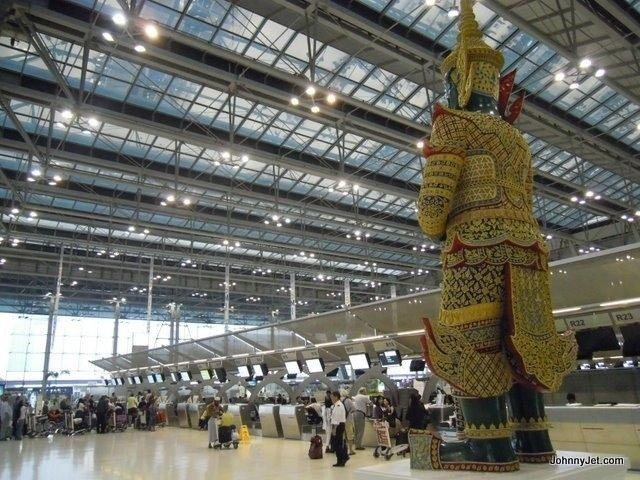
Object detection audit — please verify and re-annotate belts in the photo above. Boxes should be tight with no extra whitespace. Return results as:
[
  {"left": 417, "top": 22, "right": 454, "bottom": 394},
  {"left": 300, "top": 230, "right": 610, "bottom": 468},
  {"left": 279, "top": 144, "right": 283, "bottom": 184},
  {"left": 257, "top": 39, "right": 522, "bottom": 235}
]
[{"left": 351, "top": 410, "right": 366, "bottom": 416}]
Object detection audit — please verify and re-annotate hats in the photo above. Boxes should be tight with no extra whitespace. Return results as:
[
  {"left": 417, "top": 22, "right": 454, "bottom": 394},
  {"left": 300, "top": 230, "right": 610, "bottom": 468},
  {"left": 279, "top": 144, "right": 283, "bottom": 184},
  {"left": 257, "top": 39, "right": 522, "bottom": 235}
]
[{"left": 214, "top": 397, "right": 220, "bottom": 400}]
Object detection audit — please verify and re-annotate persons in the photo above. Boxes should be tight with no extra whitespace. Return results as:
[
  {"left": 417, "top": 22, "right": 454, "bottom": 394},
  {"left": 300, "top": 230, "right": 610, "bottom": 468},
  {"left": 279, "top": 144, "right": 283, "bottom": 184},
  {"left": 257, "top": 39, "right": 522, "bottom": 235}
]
[
  {"left": 275, "top": 386, "right": 456, "bottom": 467},
  {"left": 0, "top": 390, "right": 170, "bottom": 443},
  {"left": 407, "top": 0, "right": 579, "bottom": 473},
  {"left": 201, "top": 396, "right": 225, "bottom": 449},
  {"left": 225, "top": 397, "right": 243, "bottom": 448},
  {"left": 566, "top": 391, "right": 578, "bottom": 406}
]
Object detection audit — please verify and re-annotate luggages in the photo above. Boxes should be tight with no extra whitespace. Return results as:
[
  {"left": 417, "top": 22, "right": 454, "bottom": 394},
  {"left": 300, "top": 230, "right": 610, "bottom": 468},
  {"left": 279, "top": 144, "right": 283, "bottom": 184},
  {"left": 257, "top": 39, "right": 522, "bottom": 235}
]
[{"left": 218, "top": 425, "right": 238, "bottom": 442}]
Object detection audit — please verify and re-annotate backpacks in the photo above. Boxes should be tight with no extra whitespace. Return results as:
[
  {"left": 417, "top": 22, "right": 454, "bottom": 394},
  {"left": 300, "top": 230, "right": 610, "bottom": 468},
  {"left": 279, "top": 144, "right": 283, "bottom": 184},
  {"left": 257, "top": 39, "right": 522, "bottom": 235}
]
[
  {"left": 305, "top": 405, "right": 323, "bottom": 425},
  {"left": 308, "top": 435, "right": 323, "bottom": 459},
  {"left": 341, "top": 397, "right": 350, "bottom": 419}
]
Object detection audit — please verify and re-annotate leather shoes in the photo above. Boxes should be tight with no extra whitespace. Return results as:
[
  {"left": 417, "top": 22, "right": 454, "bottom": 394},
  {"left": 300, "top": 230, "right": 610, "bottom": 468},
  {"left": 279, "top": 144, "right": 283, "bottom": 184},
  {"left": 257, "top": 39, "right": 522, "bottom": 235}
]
[{"left": 332, "top": 456, "right": 351, "bottom": 467}]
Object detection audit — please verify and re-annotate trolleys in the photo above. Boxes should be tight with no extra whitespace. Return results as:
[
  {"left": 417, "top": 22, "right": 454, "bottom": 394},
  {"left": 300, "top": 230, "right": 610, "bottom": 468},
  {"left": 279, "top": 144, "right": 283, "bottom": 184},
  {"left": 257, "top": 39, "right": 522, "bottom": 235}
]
[
  {"left": 365, "top": 415, "right": 409, "bottom": 460},
  {"left": 213, "top": 416, "right": 239, "bottom": 450},
  {"left": 26, "top": 408, "right": 147, "bottom": 438}
]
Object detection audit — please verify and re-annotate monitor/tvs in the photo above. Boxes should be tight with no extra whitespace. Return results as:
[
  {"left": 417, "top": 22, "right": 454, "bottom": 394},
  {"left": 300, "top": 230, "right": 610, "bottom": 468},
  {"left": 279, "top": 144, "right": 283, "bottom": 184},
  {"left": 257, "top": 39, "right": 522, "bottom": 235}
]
[
  {"left": 377, "top": 350, "right": 402, "bottom": 365},
  {"left": 170, "top": 372, "right": 181, "bottom": 382},
  {"left": 348, "top": 353, "right": 371, "bottom": 369},
  {"left": 200, "top": 369, "right": 212, "bottom": 380},
  {"left": 103, "top": 373, "right": 165, "bottom": 386},
  {"left": 180, "top": 370, "right": 191, "bottom": 381},
  {"left": 574, "top": 323, "right": 640, "bottom": 352},
  {"left": 306, "top": 358, "right": 325, "bottom": 373},
  {"left": 285, "top": 360, "right": 303, "bottom": 374},
  {"left": 213, "top": 368, "right": 225, "bottom": 380},
  {"left": 252, "top": 363, "right": 268, "bottom": 376},
  {"left": 238, "top": 365, "right": 253, "bottom": 377}
]
